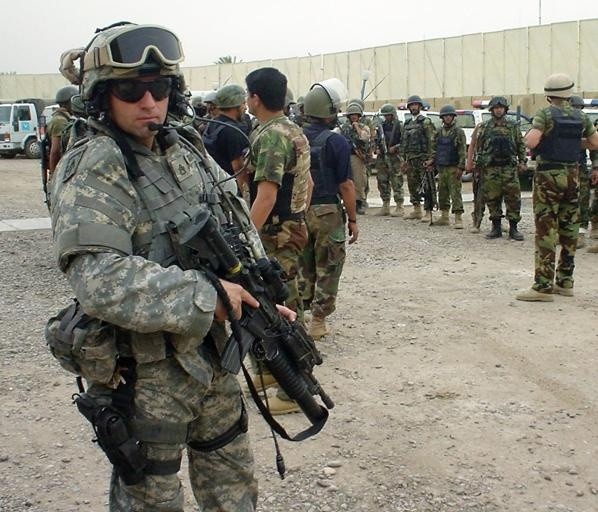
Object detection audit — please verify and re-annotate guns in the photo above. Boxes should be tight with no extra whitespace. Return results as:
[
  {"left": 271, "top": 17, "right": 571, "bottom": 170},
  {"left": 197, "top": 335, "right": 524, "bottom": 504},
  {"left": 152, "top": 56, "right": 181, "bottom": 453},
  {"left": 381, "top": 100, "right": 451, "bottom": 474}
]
[
  {"left": 336, "top": 120, "right": 371, "bottom": 158},
  {"left": 416, "top": 167, "right": 438, "bottom": 226},
  {"left": 178, "top": 210, "right": 335, "bottom": 441},
  {"left": 374, "top": 122, "right": 397, "bottom": 175},
  {"left": 38, "top": 115, "right": 47, "bottom": 194}
]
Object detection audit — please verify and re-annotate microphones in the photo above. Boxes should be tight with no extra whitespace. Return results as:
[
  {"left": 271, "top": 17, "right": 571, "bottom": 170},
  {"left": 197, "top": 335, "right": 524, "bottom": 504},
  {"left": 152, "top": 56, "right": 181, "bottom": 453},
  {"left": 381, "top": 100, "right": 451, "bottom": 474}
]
[{"left": 147, "top": 102, "right": 195, "bottom": 131}]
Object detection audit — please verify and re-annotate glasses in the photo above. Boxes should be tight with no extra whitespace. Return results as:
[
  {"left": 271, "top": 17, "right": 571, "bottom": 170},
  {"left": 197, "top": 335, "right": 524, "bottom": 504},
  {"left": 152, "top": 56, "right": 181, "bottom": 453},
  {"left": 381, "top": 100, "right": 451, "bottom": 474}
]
[
  {"left": 96, "top": 77, "right": 174, "bottom": 103},
  {"left": 83, "top": 27, "right": 185, "bottom": 72}
]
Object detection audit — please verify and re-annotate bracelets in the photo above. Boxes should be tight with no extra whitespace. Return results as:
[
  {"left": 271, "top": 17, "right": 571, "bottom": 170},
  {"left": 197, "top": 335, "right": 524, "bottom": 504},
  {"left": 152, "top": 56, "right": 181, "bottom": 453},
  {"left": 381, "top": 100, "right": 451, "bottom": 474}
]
[{"left": 348, "top": 218, "right": 356, "bottom": 223}]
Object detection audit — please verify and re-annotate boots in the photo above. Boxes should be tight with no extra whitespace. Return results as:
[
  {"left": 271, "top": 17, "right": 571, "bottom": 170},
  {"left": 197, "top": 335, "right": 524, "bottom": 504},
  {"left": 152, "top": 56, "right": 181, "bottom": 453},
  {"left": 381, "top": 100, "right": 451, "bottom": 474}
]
[
  {"left": 307, "top": 314, "right": 330, "bottom": 339},
  {"left": 256, "top": 395, "right": 301, "bottom": 415},
  {"left": 243, "top": 373, "right": 279, "bottom": 393},
  {"left": 552, "top": 282, "right": 572, "bottom": 298},
  {"left": 355, "top": 199, "right": 598, "bottom": 254},
  {"left": 517, "top": 288, "right": 553, "bottom": 302}
]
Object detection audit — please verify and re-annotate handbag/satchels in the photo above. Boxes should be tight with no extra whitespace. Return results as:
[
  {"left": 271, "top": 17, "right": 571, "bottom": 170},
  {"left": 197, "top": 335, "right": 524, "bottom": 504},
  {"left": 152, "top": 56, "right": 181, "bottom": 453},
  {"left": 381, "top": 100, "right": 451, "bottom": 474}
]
[{"left": 46, "top": 313, "right": 119, "bottom": 385}]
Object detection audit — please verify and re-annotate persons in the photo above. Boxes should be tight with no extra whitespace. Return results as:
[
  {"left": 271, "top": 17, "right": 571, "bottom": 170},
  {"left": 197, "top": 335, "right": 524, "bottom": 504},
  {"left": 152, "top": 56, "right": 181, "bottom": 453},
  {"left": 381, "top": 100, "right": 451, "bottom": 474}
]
[
  {"left": 587, "top": 117, "right": 598, "bottom": 253},
  {"left": 400, "top": 95, "right": 433, "bottom": 222},
  {"left": 202, "top": 84, "right": 249, "bottom": 198},
  {"left": 466, "top": 121, "right": 508, "bottom": 234},
  {"left": 44, "top": 85, "right": 79, "bottom": 172},
  {"left": 372, "top": 104, "right": 406, "bottom": 217},
  {"left": 296, "top": 78, "right": 358, "bottom": 337},
  {"left": 565, "top": 96, "right": 587, "bottom": 249},
  {"left": 427, "top": 104, "right": 465, "bottom": 229},
  {"left": 340, "top": 103, "right": 370, "bottom": 214},
  {"left": 191, "top": 89, "right": 304, "bottom": 136},
  {"left": 517, "top": 76, "right": 598, "bottom": 302},
  {"left": 473, "top": 96, "right": 528, "bottom": 241},
  {"left": 242, "top": 67, "right": 314, "bottom": 415}
]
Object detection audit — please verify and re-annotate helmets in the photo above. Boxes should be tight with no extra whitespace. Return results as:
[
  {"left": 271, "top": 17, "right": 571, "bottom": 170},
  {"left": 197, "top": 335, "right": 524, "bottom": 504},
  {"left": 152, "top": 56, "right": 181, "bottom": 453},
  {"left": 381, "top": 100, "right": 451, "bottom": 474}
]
[
  {"left": 55, "top": 87, "right": 78, "bottom": 103},
  {"left": 78, "top": 22, "right": 187, "bottom": 101}
]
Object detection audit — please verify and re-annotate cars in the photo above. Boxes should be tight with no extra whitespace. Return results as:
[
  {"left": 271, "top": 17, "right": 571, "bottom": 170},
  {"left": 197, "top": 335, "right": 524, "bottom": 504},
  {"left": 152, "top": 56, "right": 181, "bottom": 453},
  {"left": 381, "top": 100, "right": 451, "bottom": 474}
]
[{"left": 330, "top": 97, "right": 598, "bottom": 185}]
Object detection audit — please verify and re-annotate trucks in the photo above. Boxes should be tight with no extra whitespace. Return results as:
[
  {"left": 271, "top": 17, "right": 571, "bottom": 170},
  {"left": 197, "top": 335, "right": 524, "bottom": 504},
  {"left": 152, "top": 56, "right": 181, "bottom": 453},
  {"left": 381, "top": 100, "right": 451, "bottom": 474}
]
[{"left": 0, "top": 103, "right": 60, "bottom": 159}]
